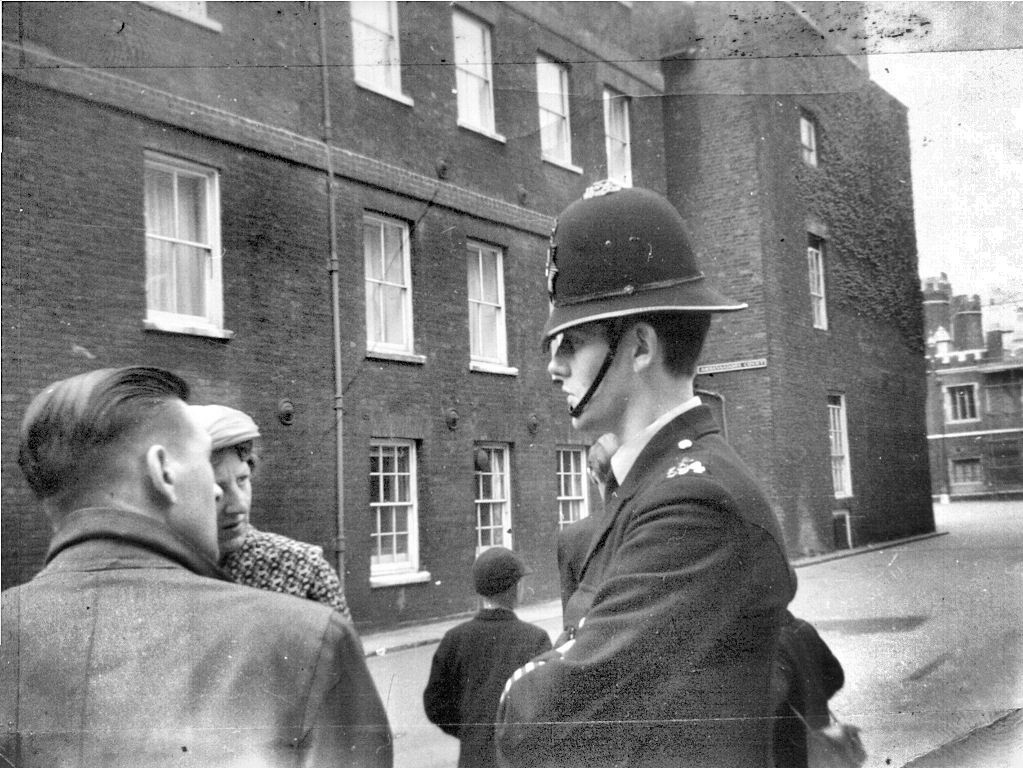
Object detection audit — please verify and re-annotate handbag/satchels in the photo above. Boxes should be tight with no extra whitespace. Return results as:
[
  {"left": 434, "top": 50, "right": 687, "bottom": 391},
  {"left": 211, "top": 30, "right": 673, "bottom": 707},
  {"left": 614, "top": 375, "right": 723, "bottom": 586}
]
[
  {"left": 804, "top": 720, "right": 866, "bottom": 768},
  {"left": 770, "top": 607, "right": 845, "bottom": 768}
]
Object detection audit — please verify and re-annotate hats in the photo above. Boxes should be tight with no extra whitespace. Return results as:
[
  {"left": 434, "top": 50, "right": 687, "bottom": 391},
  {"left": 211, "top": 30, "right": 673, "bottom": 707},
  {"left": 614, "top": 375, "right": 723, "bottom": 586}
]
[
  {"left": 471, "top": 550, "right": 535, "bottom": 599},
  {"left": 181, "top": 404, "right": 261, "bottom": 453}
]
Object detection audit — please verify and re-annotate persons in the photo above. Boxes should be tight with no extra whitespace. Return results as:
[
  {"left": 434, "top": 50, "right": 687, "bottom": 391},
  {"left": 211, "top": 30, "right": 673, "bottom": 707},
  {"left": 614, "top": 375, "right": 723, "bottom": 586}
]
[
  {"left": 1, "top": 364, "right": 393, "bottom": 768},
  {"left": 422, "top": 179, "right": 845, "bottom": 768}
]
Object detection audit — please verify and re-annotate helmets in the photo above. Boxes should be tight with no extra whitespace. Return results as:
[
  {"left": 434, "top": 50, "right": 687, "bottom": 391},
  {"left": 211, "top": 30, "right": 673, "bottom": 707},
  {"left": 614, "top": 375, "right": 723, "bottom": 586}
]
[{"left": 536, "top": 181, "right": 745, "bottom": 355}]
